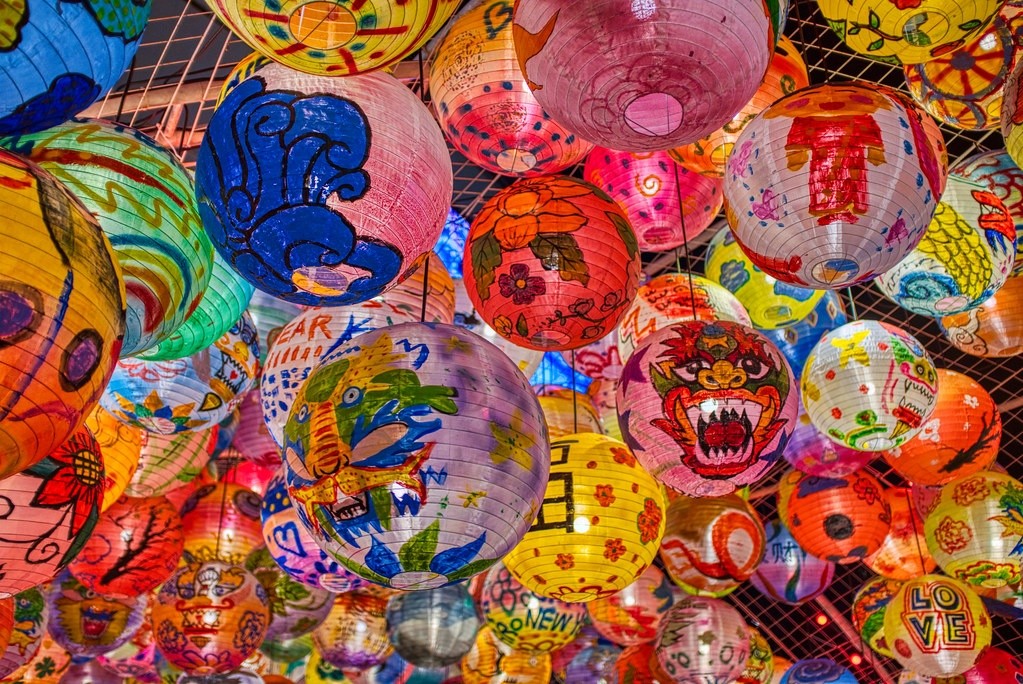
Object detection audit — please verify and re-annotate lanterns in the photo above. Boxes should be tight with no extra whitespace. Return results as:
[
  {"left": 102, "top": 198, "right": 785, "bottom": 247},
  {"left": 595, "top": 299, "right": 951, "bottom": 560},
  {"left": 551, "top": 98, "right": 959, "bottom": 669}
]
[{"left": 0, "top": 0, "right": 1023, "bottom": 684}]
